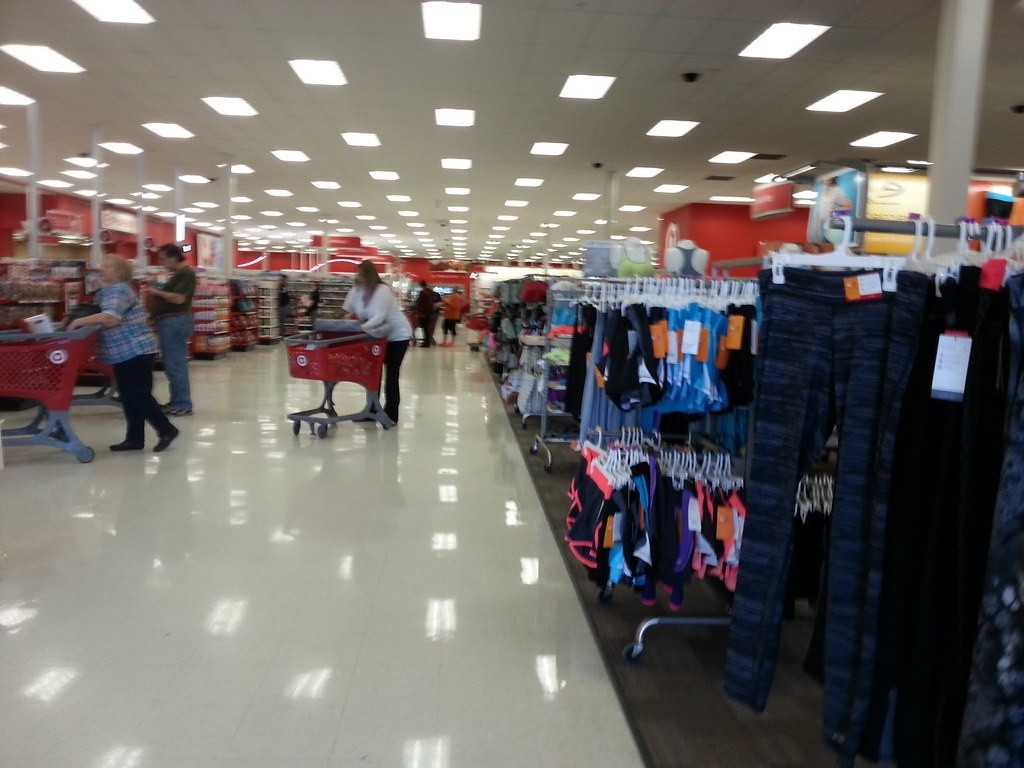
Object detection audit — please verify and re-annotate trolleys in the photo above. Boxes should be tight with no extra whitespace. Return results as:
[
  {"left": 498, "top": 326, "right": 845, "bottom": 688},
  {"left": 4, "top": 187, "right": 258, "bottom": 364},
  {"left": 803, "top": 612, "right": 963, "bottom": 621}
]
[
  {"left": 0, "top": 302, "right": 168, "bottom": 463},
  {"left": 283, "top": 313, "right": 395, "bottom": 439},
  {"left": 465, "top": 312, "right": 491, "bottom": 352},
  {"left": 400, "top": 305, "right": 436, "bottom": 347}
]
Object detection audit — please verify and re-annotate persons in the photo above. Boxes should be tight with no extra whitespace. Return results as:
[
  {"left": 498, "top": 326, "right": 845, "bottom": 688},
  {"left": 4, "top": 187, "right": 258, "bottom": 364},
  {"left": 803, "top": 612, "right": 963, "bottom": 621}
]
[
  {"left": 609, "top": 237, "right": 658, "bottom": 278},
  {"left": 340, "top": 260, "right": 414, "bottom": 427},
  {"left": 813, "top": 175, "right": 853, "bottom": 243},
  {"left": 64, "top": 253, "right": 179, "bottom": 453},
  {"left": 277, "top": 274, "right": 301, "bottom": 338},
  {"left": 139, "top": 243, "right": 194, "bottom": 417},
  {"left": 663, "top": 239, "right": 710, "bottom": 281},
  {"left": 302, "top": 281, "right": 321, "bottom": 330},
  {"left": 434, "top": 284, "right": 472, "bottom": 346},
  {"left": 409, "top": 280, "right": 444, "bottom": 348}
]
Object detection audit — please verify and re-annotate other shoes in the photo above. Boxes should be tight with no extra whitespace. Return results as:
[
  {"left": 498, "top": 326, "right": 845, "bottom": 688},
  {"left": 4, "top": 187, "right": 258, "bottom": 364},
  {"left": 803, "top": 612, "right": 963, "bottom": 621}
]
[
  {"left": 152, "top": 425, "right": 179, "bottom": 452},
  {"left": 352, "top": 412, "right": 377, "bottom": 422},
  {"left": 384, "top": 412, "right": 398, "bottom": 424},
  {"left": 110, "top": 438, "right": 145, "bottom": 450}
]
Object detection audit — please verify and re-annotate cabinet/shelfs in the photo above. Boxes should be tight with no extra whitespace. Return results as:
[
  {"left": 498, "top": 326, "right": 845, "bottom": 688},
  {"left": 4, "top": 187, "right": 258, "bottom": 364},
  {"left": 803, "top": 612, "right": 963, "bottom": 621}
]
[{"left": 0, "top": 257, "right": 581, "bottom": 467}]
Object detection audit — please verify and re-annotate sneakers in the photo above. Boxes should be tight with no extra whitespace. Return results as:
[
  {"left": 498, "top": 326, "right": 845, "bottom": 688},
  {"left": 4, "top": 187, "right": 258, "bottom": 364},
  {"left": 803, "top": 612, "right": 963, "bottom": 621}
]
[{"left": 159, "top": 402, "right": 193, "bottom": 417}]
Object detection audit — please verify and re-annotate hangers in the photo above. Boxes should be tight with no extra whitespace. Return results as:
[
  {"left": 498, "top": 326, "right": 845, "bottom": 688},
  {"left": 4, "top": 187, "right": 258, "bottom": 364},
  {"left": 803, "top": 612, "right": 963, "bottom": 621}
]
[{"left": 571, "top": 212, "right": 1024, "bottom": 526}]
